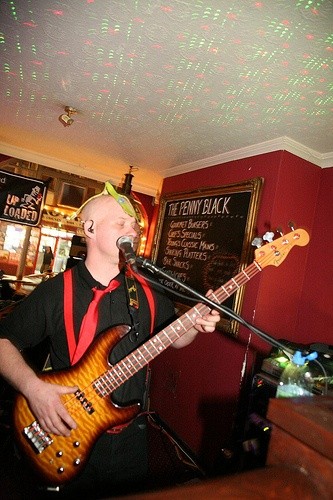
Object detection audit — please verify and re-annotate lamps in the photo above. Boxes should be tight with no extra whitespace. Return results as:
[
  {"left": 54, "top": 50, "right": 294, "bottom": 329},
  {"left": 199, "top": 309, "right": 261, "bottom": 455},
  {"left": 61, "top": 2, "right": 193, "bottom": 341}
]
[{"left": 58, "top": 105, "right": 80, "bottom": 128}]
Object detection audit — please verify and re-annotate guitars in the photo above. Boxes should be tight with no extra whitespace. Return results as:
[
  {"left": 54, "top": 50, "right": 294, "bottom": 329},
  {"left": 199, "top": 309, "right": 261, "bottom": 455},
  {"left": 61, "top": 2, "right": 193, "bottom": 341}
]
[{"left": 13, "top": 222, "right": 310, "bottom": 481}]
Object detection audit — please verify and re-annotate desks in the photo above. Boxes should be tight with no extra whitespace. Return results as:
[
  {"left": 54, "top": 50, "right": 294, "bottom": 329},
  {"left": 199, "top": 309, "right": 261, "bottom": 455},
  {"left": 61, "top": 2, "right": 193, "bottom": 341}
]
[{"left": 265, "top": 394, "right": 333, "bottom": 500}]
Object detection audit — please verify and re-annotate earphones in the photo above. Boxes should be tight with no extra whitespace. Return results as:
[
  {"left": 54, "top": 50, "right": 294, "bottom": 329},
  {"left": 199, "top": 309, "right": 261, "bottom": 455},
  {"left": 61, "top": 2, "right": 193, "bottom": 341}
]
[{"left": 88, "top": 227, "right": 94, "bottom": 233}]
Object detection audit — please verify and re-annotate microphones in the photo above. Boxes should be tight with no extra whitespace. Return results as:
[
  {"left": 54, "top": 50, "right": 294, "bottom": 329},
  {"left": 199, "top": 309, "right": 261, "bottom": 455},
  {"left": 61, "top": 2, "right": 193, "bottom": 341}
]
[{"left": 116, "top": 235, "right": 137, "bottom": 264}]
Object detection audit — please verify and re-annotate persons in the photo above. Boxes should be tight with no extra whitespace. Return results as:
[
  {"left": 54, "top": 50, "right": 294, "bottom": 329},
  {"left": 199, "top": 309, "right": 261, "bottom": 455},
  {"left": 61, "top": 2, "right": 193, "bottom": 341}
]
[
  {"left": 0, "top": 195, "right": 221, "bottom": 500},
  {"left": 132, "top": 223, "right": 142, "bottom": 252},
  {"left": 40, "top": 246, "right": 54, "bottom": 280}
]
